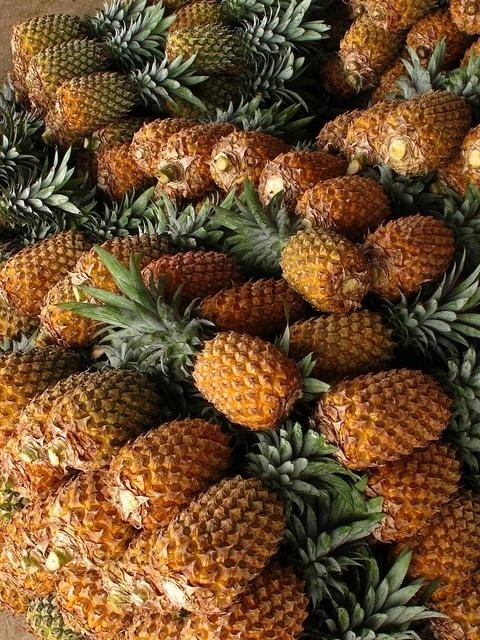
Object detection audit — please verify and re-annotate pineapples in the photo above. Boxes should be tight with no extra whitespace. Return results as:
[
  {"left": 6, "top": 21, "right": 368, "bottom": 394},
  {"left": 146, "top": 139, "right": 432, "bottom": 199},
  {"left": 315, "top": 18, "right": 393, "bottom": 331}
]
[{"left": 0, "top": 0, "right": 480, "bottom": 640}]
